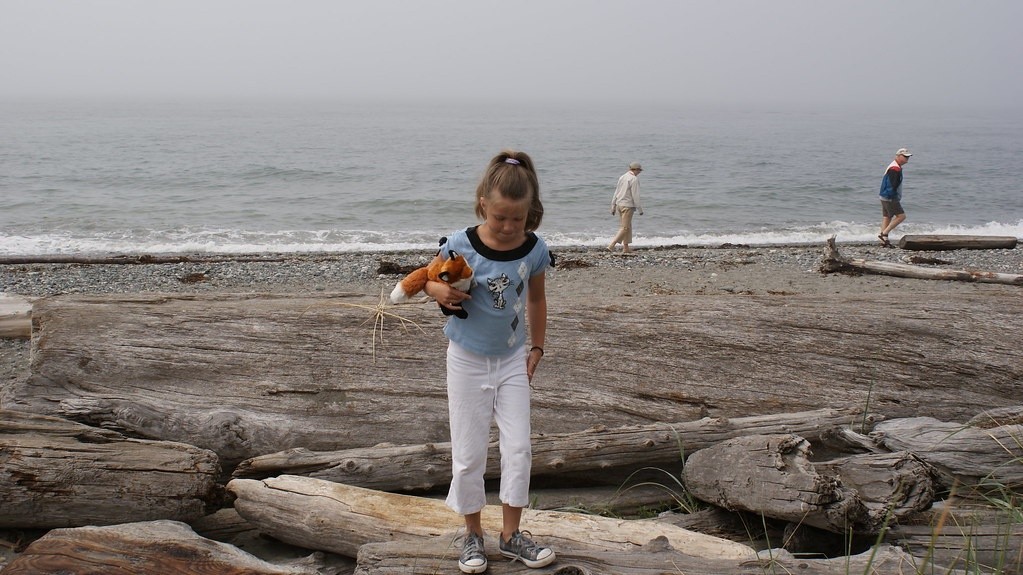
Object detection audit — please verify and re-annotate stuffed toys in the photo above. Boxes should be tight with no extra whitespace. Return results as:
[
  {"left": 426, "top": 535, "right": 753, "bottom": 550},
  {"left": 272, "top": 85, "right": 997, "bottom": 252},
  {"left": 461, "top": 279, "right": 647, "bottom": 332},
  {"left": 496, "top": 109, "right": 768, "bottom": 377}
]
[{"left": 391, "top": 251, "right": 477, "bottom": 319}]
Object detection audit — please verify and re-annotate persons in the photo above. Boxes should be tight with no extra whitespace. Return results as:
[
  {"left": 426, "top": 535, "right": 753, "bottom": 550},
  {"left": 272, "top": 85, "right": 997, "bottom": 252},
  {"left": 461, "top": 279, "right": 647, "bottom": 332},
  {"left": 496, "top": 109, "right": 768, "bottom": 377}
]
[
  {"left": 608, "top": 162, "right": 643, "bottom": 254},
  {"left": 423, "top": 152, "right": 556, "bottom": 572},
  {"left": 879, "top": 148, "right": 913, "bottom": 249}
]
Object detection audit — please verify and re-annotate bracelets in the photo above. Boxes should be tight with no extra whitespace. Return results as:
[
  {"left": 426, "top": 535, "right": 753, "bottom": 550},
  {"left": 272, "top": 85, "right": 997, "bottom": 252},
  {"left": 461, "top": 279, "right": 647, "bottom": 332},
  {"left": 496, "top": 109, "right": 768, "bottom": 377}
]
[
  {"left": 530, "top": 346, "right": 543, "bottom": 356},
  {"left": 529, "top": 361, "right": 536, "bottom": 366}
]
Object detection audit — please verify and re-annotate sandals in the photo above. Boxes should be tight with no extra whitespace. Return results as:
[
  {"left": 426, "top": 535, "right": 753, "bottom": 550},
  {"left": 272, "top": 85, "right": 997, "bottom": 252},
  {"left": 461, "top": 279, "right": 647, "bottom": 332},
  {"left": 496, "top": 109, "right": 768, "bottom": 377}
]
[
  {"left": 879, "top": 242, "right": 897, "bottom": 248},
  {"left": 878, "top": 233, "right": 890, "bottom": 245}
]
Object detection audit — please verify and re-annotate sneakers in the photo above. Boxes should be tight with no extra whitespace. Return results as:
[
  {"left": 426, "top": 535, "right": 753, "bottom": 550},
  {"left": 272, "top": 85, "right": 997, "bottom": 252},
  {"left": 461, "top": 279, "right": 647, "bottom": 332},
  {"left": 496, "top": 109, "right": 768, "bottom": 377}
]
[
  {"left": 498, "top": 529, "right": 556, "bottom": 568},
  {"left": 453, "top": 531, "right": 488, "bottom": 574}
]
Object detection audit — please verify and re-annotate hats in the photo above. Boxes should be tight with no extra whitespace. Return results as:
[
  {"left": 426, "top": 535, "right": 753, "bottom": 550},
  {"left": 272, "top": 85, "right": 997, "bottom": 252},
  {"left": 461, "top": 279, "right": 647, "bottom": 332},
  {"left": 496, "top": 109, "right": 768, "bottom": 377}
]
[
  {"left": 629, "top": 162, "right": 644, "bottom": 172},
  {"left": 895, "top": 148, "right": 913, "bottom": 157}
]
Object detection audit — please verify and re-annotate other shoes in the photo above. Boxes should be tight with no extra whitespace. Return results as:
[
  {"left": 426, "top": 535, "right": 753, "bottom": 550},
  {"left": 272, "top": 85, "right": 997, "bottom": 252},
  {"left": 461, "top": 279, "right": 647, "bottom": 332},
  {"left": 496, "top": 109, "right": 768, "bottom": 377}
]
[
  {"left": 623, "top": 249, "right": 634, "bottom": 254},
  {"left": 607, "top": 245, "right": 618, "bottom": 254}
]
